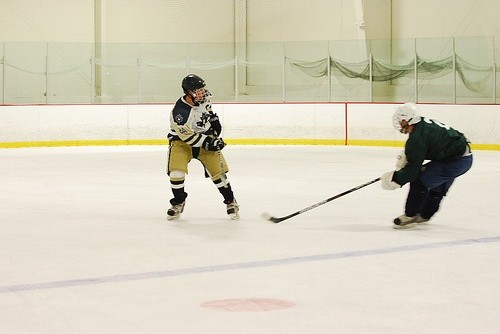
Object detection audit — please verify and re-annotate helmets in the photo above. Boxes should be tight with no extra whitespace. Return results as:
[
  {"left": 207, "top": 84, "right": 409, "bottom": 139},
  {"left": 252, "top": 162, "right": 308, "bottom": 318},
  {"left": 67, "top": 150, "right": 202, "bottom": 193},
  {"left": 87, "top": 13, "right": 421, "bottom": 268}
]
[
  {"left": 182, "top": 73, "right": 206, "bottom": 91},
  {"left": 392, "top": 105, "right": 421, "bottom": 134}
]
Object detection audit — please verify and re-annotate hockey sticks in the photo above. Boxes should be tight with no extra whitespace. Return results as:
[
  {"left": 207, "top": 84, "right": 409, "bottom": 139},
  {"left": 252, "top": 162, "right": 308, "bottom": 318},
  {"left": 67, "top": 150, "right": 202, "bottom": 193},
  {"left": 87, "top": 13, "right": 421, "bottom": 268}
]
[
  {"left": 262, "top": 177, "right": 382, "bottom": 223},
  {"left": 213, "top": 129, "right": 229, "bottom": 189}
]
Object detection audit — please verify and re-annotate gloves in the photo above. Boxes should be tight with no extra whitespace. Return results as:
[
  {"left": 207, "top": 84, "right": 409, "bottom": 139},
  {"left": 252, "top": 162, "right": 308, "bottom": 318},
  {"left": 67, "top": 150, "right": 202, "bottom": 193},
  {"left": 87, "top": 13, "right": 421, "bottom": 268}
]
[
  {"left": 202, "top": 135, "right": 226, "bottom": 151},
  {"left": 395, "top": 153, "right": 407, "bottom": 171},
  {"left": 209, "top": 113, "right": 222, "bottom": 136},
  {"left": 379, "top": 170, "right": 402, "bottom": 190}
]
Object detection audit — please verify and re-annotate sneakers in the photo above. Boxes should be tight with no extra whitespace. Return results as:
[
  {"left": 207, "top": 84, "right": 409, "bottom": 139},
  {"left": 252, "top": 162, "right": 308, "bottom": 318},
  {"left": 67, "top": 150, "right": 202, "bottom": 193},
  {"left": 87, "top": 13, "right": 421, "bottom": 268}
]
[
  {"left": 167, "top": 201, "right": 185, "bottom": 220},
  {"left": 393, "top": 214, "right": 416, "bottom": 229},
  {"left": 415, "top": 213, "right": 429, "bottom": 225},
  {"left": 223, "top": 197, "right": 240, "bottom": 219}
]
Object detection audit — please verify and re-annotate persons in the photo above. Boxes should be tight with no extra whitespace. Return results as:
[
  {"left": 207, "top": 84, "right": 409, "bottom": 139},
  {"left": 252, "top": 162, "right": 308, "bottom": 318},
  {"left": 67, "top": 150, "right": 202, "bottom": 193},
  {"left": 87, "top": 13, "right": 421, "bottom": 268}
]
[
  {"left": 380, "top": 104, "right": 473, "bottom": 229},
  {"left": 167, "top": 74, "right": 240, "bottom": 221}
]
